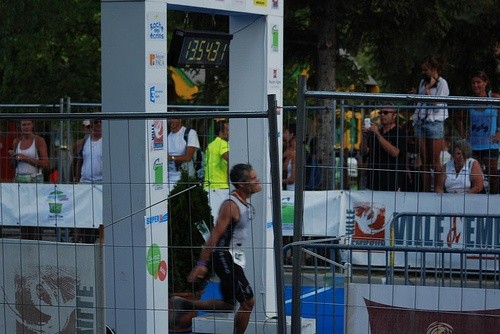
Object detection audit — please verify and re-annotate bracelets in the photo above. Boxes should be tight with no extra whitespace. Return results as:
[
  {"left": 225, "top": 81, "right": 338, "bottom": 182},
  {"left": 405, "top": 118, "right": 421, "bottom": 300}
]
[
  {"left": 197, "top": 261, "right": 207, "bottom": 267},
  {"left": 171, "top": 155, "right": 175, "bottom": 162}
]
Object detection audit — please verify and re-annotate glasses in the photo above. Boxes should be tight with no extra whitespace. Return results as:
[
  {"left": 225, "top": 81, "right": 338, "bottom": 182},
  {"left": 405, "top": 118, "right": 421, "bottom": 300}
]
[
  {"left": 94, "top": 120, "right": 101, "bottom": 124},
  {"left": 378, "top": 110, "right": 397, "bottom": 115},
  {"left": 86, "top": 125, "right": 92, "bottom": 129}
]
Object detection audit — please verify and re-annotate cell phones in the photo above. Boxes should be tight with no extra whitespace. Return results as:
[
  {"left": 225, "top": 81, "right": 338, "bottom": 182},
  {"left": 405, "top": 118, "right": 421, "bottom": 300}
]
[{"left": 365, "top": 118, "right": 370, "bottom": 129}]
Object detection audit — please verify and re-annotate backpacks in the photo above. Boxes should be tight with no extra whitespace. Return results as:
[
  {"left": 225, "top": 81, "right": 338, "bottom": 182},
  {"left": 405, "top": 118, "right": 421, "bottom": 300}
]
[{"left": 167, "top": 127, "right": 202, "bottom": 171}]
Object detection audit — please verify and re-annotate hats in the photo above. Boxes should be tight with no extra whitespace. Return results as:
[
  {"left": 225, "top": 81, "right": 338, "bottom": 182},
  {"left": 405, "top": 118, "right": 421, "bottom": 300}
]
[{"left": 82, "top": 120, "right": 90, "bottom": 126}]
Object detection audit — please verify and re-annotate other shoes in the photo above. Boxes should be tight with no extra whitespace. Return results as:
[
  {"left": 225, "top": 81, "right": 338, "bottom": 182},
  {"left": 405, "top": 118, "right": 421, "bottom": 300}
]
[{"left": 171, "top": 295, "right": 183, "bottom": 326}]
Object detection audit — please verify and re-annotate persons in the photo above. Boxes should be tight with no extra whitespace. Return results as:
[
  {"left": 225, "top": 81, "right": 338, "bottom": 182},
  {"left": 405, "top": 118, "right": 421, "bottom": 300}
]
[
  {"left": 10, "top": 55, "right": 500, "bottom": 195},
  {"left": 171, "top": 164, "right": 261, "bottom": 334}
]
